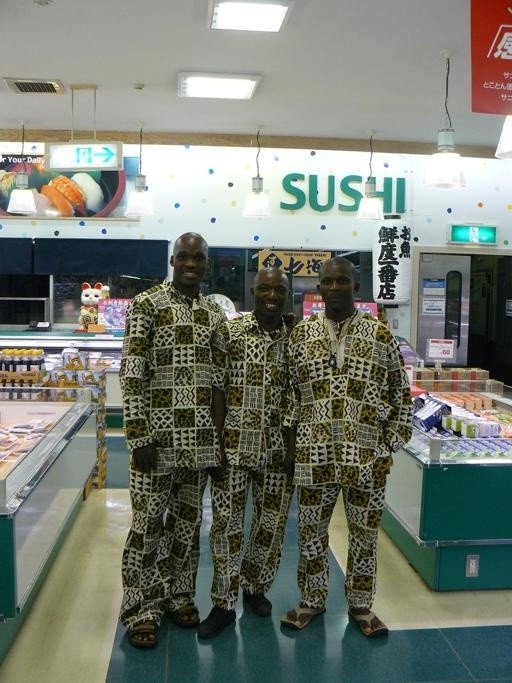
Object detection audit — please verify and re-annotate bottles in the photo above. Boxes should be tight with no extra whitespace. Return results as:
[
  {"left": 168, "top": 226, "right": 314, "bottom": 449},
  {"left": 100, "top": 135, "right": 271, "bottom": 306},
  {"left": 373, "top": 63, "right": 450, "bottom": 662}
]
[
  {"left": 0, "top": 378, "right": 3, "bottom": 400},
  {"left": 5, "top": 379, "right": 12, "bottom": 399},
  {"left": 31, "top": 379, "right": 40, "bottom": 400},
  {"left": 14, "top": 380, "right": 22, "bottom": 399},
  {"left": 0, "top": 348, "right": 45, "bottom": 372},
  {"left": 21, "top": 379, "right": 31, "bottom": 399}
]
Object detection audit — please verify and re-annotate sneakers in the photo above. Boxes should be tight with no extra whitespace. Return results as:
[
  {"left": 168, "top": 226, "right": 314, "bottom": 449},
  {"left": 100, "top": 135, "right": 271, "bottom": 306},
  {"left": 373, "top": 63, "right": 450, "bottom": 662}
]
[
  {"left": 197, "top": 606, "right": 236, "bottom": 639},
  {"left": 242, "top": 590, "right": 272, "bottom": 618}
]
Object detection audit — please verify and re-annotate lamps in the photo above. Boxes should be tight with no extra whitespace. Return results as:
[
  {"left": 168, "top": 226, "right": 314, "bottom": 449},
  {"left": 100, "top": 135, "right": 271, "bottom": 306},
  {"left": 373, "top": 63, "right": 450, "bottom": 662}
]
[
  {"left": 246, "top": 128, "right": 271, "bottom": 219},
  {"left": 495, "top": 116, "right": 512, "bottom": 164},
  {"left": 360, "top": 128, "right": 382, "bottom": 222},
  {"left": 124, "top": 127, "right": 154, "bottom": 216},
  {"left": 430, "top": 49, "right": 464, "bottom": 199},
  {"left": 8, "top": 124, "right": 38, "bottom": 214}
]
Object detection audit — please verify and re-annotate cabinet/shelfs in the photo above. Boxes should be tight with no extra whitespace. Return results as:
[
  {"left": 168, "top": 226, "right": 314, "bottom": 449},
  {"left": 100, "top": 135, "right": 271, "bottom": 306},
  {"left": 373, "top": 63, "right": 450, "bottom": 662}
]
[
  {"left": 1, "top": 386, "right": 97, "bottom": 663},
  {"left": 2, "top": 331, "right": 127, "bottom": 428},
  {"left": 378, "top": 375, "right": 512, "bottom": 592}
]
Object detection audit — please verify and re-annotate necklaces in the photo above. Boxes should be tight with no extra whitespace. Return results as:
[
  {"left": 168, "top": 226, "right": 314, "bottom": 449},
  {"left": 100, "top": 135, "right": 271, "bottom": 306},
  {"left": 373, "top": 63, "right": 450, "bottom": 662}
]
[{"left": 323, "top": 311, "right": 358, "bottom": 366}]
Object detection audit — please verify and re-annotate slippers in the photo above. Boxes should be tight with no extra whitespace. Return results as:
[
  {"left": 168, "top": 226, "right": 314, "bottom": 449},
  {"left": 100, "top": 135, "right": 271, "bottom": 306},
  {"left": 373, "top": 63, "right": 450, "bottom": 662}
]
[
  {"left": 280, "top": 604, "right": 326, "bottom": 630},
  {"left": 347, "top": 608, "right": 389, "bottom": 637},
  {"left": 129, "top": 621, "right": 159, "bottom": 648},
  {"left": 168, "top": 606, "right": 200, "bottom": 628}
]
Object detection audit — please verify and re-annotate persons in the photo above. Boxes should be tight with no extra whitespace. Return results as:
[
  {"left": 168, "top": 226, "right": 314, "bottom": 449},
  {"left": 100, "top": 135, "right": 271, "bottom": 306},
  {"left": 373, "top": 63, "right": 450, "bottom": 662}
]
[
  {"left": 281, "top": 256, "right": 412, "bottom": 638},
  {"left": 197, "top": 267, "right": 298, "bottom": 638},
  {"left": 122, "top": 233, "right": 297, "bottom": 648}
]
